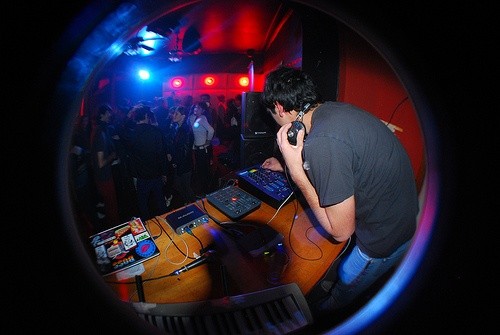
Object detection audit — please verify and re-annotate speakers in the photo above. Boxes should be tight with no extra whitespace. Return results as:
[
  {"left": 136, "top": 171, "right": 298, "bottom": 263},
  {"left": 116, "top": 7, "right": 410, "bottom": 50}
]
[{"left": 239, "top": 91, "right": 286, "bottom": 169}]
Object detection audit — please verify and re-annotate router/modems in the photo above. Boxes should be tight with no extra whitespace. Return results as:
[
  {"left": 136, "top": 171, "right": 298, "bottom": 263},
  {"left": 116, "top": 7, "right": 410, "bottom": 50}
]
[{"left": 166, "top": 204, "right": 209, "bottom": 234}]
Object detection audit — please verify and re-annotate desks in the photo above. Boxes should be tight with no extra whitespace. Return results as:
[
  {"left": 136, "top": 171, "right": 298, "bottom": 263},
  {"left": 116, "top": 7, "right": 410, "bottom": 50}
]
[{"left": 83, "top": 185, "right": 349, "bottom": 305}]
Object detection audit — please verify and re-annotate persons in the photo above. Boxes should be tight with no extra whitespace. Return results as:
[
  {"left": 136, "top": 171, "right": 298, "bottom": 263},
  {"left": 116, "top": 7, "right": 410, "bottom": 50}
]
[
  {"left": 66, "top": 93, "right": 242, "bottom": 228},
  {"left": 261, "top": 68, "right": 421, "bottom": 323}
]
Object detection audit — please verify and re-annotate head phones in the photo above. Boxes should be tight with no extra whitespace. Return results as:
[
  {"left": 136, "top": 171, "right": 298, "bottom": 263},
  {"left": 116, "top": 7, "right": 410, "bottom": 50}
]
[{"left": 287, "top": 98, "right": 318, "bottom": 146}]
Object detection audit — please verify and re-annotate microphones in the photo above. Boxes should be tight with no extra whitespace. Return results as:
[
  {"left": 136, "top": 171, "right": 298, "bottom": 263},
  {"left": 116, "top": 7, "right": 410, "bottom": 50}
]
[{"left": 171, "top": 249, "right": 217, "bottom": 276}]
